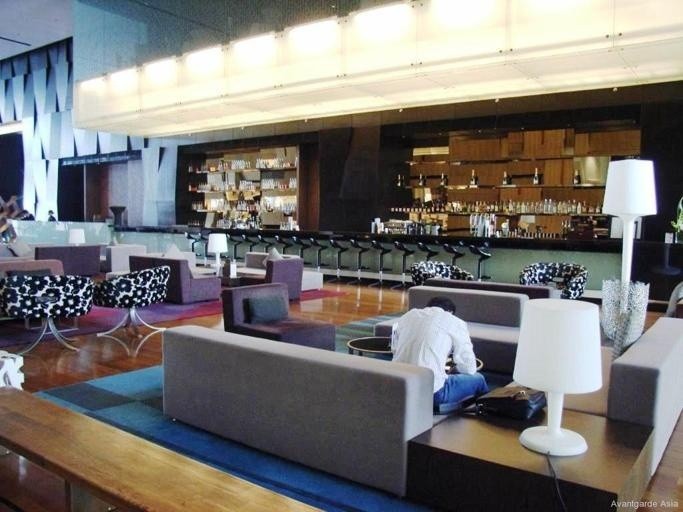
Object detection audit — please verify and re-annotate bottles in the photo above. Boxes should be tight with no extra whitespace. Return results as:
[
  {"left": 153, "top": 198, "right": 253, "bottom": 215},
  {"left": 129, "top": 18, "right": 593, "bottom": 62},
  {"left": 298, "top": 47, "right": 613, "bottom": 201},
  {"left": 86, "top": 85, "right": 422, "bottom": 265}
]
[
  {"left": 439, "top": 172, "right": 446, "bottom": 185},
  {"left": 502, "top": 171, "right": 509, "bottom": 184},
  {"left": 390, "top": 198, "right": 602, "bottom": 215},
  {"left": 532, "top": 168, "right": 539, "bottom": 185},
  {"left": 188, "top": 155, "right": 297, "bottom": 230},
  {"left": 469, "top": 168, "right": 477, "bottom": 185},
  {"left": 560, "top": 219, "right": 570, "bottom": 239},
  {"left": 572, "top": 169, "right": 580, "bottom": 185},
  {"left": 222, "top": 258, "right": 236, "bottom": 277},
  {"left": 418, "top": 172, "right": 424, "bottom": 187},
  {"left": 396, "top": 174, "right": 401, "bottom": 186},
  {"left": 468, "top": 213, "right": 510, "bottom": 236}
]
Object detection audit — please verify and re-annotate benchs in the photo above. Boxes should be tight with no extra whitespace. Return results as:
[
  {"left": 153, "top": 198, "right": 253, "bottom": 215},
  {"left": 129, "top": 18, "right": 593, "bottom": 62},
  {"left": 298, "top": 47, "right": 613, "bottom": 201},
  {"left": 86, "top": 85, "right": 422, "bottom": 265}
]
[
  {"left": 252, "top": 254, "right": 304, "bottom": 302},
  {"left": 0, "top": 381, "right": 329, "bottom": 510},
  {"left": 131, "top": 254, "right": 223, "bottom": 304},
  {"left": 101, "top": 242, "right": 147, "bottom": 272},
  {"left": 33, "top": 244, "right": 102, "bottom": 276},
  {"left": 238, "top": 250, "right": 299, "bottom": 279},
  {"left": 0, "top": 259, "right": 67, "bottom": 317}
]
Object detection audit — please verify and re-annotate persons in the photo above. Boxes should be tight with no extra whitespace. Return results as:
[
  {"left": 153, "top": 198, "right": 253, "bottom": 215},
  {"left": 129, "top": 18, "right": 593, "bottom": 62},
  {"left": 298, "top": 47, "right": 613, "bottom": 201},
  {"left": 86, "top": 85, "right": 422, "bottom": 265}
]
[
  {"left": 48, "top": 210, "right": 56, "bottom": 221},
  {"left": 392, "top": 296, "right": 489, "bottom": 414}
]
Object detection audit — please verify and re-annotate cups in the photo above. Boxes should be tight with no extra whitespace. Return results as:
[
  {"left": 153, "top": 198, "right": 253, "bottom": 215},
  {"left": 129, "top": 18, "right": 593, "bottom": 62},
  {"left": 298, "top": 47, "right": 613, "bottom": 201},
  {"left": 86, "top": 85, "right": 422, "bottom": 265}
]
[
  {"left": 508, "top": 229, "right": 558, "bottom": 238},
  {"left": 370, "top": 222, "right": 440, "bottom": 233}
]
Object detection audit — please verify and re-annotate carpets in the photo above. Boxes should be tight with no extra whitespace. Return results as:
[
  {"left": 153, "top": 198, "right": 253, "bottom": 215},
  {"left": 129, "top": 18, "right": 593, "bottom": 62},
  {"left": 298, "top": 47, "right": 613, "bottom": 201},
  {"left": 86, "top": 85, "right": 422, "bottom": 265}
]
[{"left": 26, "top": 307, "right": 519, "bottom": 511}]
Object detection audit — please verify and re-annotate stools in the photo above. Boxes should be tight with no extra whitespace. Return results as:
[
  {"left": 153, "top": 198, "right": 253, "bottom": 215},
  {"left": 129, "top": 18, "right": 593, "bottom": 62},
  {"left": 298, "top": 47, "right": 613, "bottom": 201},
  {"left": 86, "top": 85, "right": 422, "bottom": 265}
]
[
  {"left": 329, "top": 240, "right": 348, "bottom": 267},
  {"left": 199, "top": 233, "right": 214, "bottom": 256},
  {"left": 350, "top": 239, "right": 371, "bottom": 269},
  {"left": 371, "top": 240, "right": 392, "bottom": 272},
  {"left": 184, "top": 232, "right": 200, "bottom": 251},
  {"left": 275, "top": 237, "right": 293, "bottom": 254},
  {"left": 310, "top": 238, "right": 328, "bottom": 266},
  {"left": 468, "top": 242, "right": 493, "bottom": 280},
  {"left": 394, "top": 241, "right": 414, "bottom": 274},
  {"left": 418, "top": 242, "right": 440, "bottom": 260},
  {"left": 242, "top": 235, "right": 259, "bottom": 253},
  {"left": 443, "top": 242, "right": 467, "bottom": 266},
  {"left": 289, "top": 236, "right": 311, "bottom": 257},
  {"left": 255, "top": 235, "right": 276, "bottom": 252},
  {"left": 226, "top": 235, "right": 242, "bottom": 260}
]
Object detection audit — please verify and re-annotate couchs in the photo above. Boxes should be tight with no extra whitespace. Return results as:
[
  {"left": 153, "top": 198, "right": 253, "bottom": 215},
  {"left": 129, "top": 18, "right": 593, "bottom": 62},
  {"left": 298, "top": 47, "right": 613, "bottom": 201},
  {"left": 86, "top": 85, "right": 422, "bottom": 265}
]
[
  {"left": 421, "top": 275, "right": 556, "bottom": 302},
  {"left": 366, "top": 281, "right": 533, "bottom": 388},
  {"left": 151, "top": 319, "right": 462, "bottom": 498},
  {"left": 493, "top": 312, "right": 682, "bottom": 481}
]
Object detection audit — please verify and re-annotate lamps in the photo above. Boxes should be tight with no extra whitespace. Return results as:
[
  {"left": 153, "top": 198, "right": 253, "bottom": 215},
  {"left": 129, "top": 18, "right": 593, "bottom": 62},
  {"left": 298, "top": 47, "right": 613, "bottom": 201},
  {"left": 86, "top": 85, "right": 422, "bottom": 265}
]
[
  {"left": 501, "top": 293, "right": 608, "bottom": 462},
  {"left": 598, "top": 154, "right": 661, "bottom": 315}
]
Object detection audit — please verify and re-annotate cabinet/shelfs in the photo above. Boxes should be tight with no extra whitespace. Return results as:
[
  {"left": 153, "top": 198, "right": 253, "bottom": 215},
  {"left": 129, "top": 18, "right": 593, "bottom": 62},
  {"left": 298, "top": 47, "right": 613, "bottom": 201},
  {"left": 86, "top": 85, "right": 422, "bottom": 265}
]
[
  {"left": 172, "top": 133, "right": 321, "bottom": 233},
  {"left": 392, "top": 152, "right": 643, "bottom": 239}
]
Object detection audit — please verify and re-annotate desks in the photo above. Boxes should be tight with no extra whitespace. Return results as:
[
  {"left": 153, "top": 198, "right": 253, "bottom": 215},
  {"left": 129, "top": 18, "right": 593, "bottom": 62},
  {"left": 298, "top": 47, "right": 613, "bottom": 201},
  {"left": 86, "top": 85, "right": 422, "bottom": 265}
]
[{"left": 401, "top": 395, "right": 658, "bottom": 512}]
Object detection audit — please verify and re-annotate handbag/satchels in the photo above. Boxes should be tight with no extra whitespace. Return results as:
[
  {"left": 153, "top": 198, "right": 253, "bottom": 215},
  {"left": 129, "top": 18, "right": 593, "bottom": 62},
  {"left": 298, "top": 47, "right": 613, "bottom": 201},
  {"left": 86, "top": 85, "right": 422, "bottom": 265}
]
[{"left": 474, "top": 384, "right": 548, "bottom": 424}]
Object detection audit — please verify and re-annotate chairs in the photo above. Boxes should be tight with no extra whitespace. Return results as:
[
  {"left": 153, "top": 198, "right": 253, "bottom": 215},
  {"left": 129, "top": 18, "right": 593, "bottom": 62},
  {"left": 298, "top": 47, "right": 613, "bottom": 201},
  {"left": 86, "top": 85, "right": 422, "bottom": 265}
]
[
  {"left": 0, "top": 272, "right": 93, "bottom": 356},
  {"left": 93, "top": 265, "right": 165, "bottom": 355},
  {"left": 517, "top": 260, "right": 591, "bottom": 302}
]
